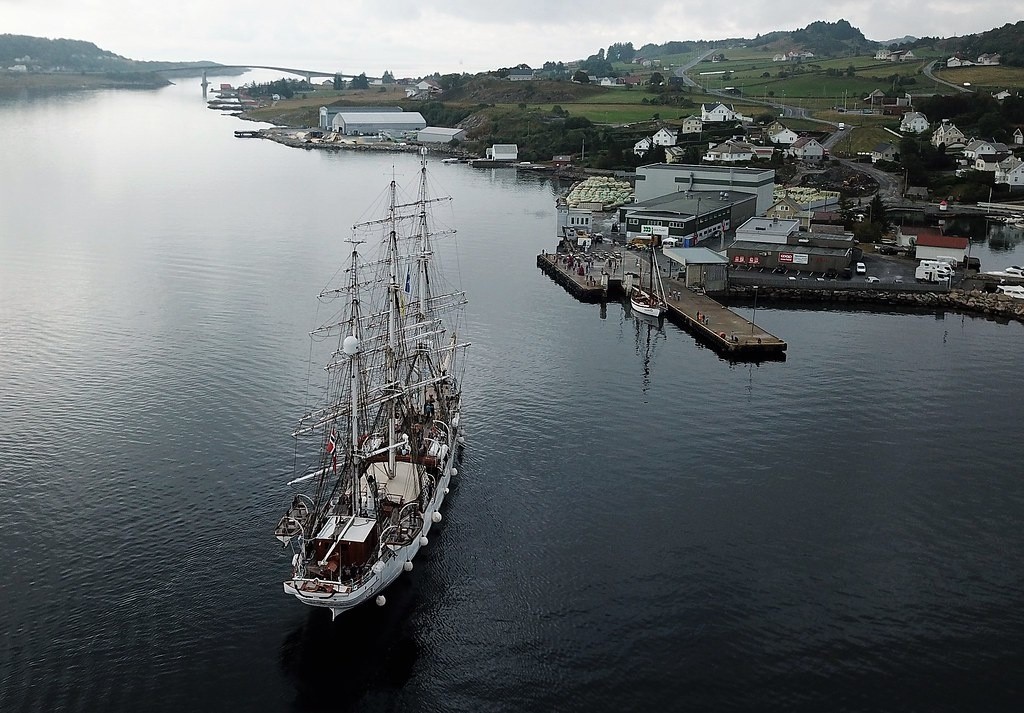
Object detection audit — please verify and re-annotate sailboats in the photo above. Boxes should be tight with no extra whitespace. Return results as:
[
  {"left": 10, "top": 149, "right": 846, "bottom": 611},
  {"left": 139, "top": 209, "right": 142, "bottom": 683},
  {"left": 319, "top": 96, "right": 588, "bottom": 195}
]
[
  {"left": 629, "top": 229, "right": 669, "bottom": 318},
  {"left": 628, "top": 309, "right": 665, "bottom": 394},
  {"left": 272, "top": 146, "right": 473, "bottom": 623}
]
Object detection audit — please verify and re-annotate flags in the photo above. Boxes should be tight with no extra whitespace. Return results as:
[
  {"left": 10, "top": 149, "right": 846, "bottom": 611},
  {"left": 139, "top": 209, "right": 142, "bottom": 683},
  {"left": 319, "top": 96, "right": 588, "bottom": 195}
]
[{"left": 326, "top": 429, "right": 338, "bottom": 475}]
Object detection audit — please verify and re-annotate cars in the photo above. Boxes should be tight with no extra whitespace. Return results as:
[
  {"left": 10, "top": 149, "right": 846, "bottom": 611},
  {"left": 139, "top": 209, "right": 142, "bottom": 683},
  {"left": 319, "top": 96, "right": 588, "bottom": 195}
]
[
  {"left": 856, "top": 262, "right": 867, "bottom": 275},
  {"left": 864, "top": 276, "right": 880, "bottom": 283}
]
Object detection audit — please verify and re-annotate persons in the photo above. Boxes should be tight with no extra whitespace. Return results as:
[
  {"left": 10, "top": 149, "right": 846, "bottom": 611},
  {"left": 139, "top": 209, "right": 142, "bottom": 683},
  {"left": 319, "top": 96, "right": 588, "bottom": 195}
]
[
  {"left": 756, "top": 337, "right": 762, "bottom": 345},
  {"left": 424, "top": 392, "right": 435, "bottom": 421},
  {"left": 668, "top": 287, "right": 682, "bottom": 301},
  {"left": 719, "top": 330, "right": 738, "bottom": 343},
  {"left": 695, "top": 309, "right": 709, "bottom": 325}
]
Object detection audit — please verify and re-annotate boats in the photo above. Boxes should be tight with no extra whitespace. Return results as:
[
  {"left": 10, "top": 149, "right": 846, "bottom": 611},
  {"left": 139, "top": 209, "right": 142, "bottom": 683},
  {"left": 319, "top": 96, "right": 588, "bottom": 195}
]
[
  {"left": 983, "top": 265, "right": 1024, "bottom": 279},
  {"left": 275, "top": 493, "right": 316, "bottom": 548},
  {"left": 994, "top": 284, "right": 1024, "bottom": 301}
]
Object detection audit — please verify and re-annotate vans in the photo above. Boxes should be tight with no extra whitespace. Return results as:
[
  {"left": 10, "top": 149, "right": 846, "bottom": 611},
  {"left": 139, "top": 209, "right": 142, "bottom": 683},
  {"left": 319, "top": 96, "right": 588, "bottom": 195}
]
[{"left": 915, "top": 259, "right": 956, "bottom": 284}]
